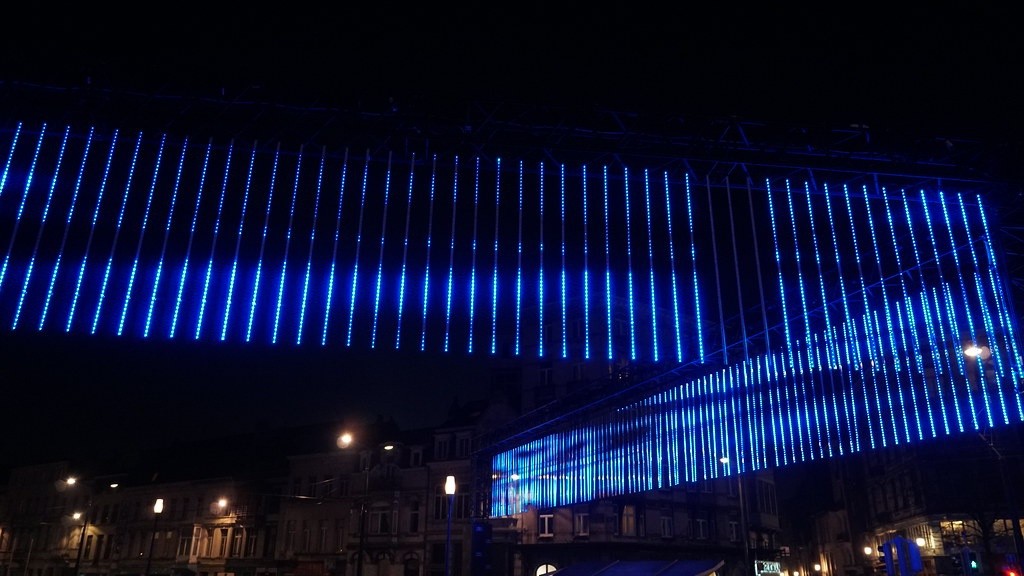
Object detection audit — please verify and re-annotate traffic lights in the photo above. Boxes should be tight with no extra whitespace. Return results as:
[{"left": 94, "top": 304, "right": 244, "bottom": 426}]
[
  {"left": 970, "top": 560, "right": 978, "bottom": 569},
  {"left": 1006, "top": 570, "right": 1018, "bottom": 576}
]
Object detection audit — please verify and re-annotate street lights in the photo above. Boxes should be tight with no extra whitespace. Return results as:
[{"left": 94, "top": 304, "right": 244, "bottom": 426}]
[
  {"left": 65, "top": 475, "right": 119, "bottom": 576},
  {"left": 145, "top": 495, "right": 164, "bottom": 576},
  {"left": 719, "top": 457, "right": 753, "bottom": 576},
  {"left": 444, "top": 475, "right": 456, "bottom": 576},
  {"left": 337, "top": 432, "right": 395, "bottom": 576},
  {"left": 962, "top": 343, "right": 1024, "bottom": 575}
]
